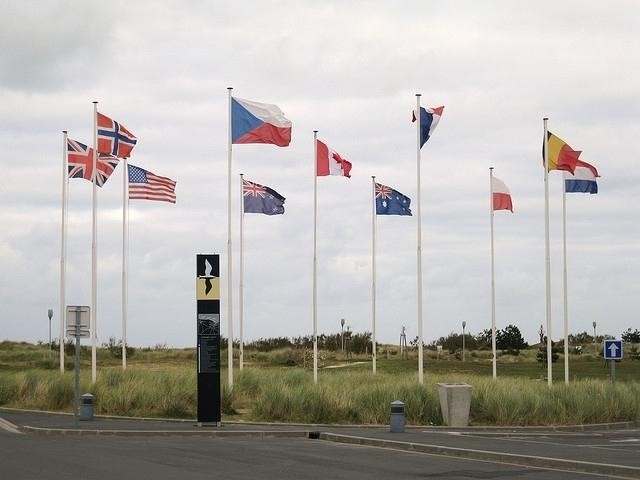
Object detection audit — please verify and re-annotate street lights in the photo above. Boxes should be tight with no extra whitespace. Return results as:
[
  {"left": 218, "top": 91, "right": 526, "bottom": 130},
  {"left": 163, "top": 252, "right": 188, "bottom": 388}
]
[
  {"left": 593, "top": 321, "right": 597, "bottom": 354},
  {"left": 462, "top": 321, "right": 466, "bottom": 363},
  {"left": 340, "top": 319, "right": 345, "bottom": 353},
  {"left": 48, "top": 308, "right": 54, "bottom": 362}
]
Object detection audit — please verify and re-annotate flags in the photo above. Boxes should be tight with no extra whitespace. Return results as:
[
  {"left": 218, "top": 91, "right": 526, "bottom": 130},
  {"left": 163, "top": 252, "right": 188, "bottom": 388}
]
[
  {"left": 231, "top": 96, "right": 293, "bottom": 147},
  {"left": 126, "top": 163, "right": 177, "bottom": 205},
  {"left": 316, "top": 138, "right": 352, "bottom": 179},
  {"left": 491, "top": 176, "right": 513, "bottom": 214},
  {"left": 243, "top": 179, "right": 285, "bottom": 216},
  {"left": 96, "top": 110, "right": 138, "bottom": 159},
  {"left": 374, "top": 182, "right": 413, "bottom": 217},
  {"left": 66, "top": 138, "right": 121, "bottom": 189},
  {"left": 411, "top": 105, "right": 446, "bottom": 149},
  {"left": 565, "top": 157, "right": 603, "bottom": 194},
  {"left": 542, "top": 130, "right": 582, "bottom": 176}
]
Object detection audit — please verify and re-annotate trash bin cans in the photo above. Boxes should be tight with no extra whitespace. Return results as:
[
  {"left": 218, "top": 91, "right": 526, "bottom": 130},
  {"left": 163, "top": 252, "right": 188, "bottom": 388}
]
[
  {"left": 79, "top": 392, "right": 97, "bottom": 420},
  {"left": 389, "top": 400, "right": 406, "bottom": 433},
  {"left": 437, "top": 382, "right": 473, "bottom": 426}
]
